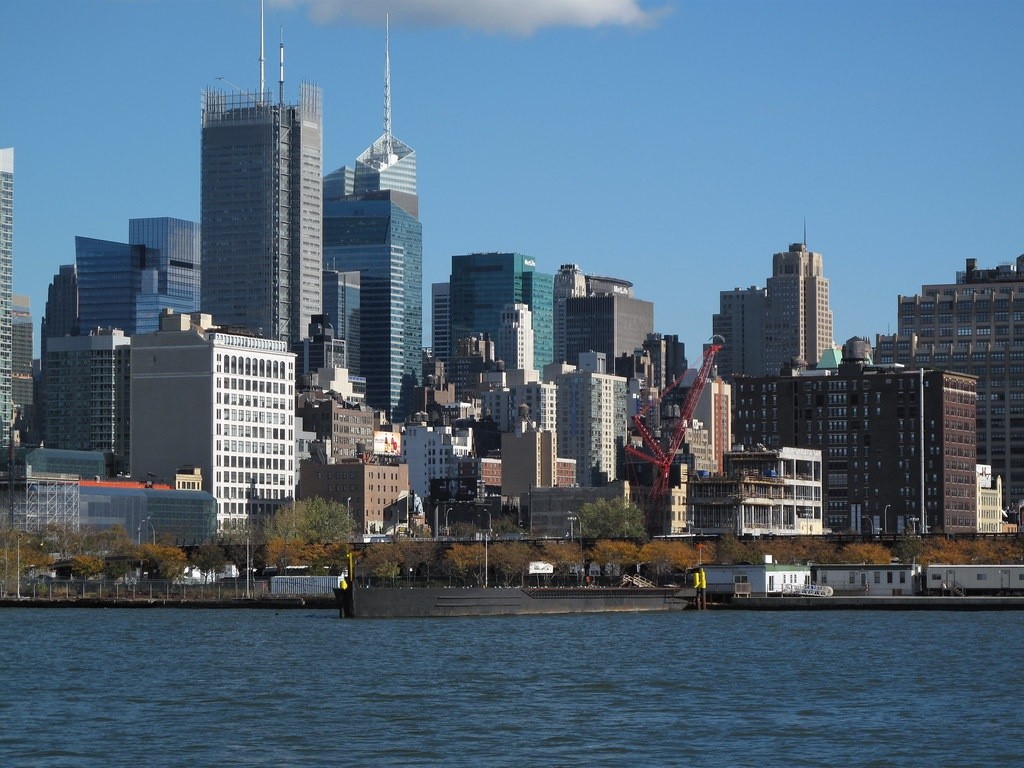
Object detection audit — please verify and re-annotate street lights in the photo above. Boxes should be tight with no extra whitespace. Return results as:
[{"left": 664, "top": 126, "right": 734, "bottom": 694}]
[
  {"left": 484, "top": 509, "right": 493, "bottom": 531},
  {"left": 17, "top": 538, "right": 22, "bottom": 598},
  {"left": 446, "top": 507, "right": 454, "bottom": 528},
  {"left": 137, "top": 516, "right": 155, "bottom": 545},
  {"left": 347, "top": 497, "right": 353, "bottom": 525},
  {"left": 884, "top": 505, "right": 891, "bottom": 532},
  {"left": 567, "top": 511, "right": 582, "bottom": 536}
]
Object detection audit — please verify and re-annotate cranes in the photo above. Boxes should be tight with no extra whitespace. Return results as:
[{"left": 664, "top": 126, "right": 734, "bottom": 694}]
[{"left": 622, "top": 342, "right": 724, "bottom": 504}]
